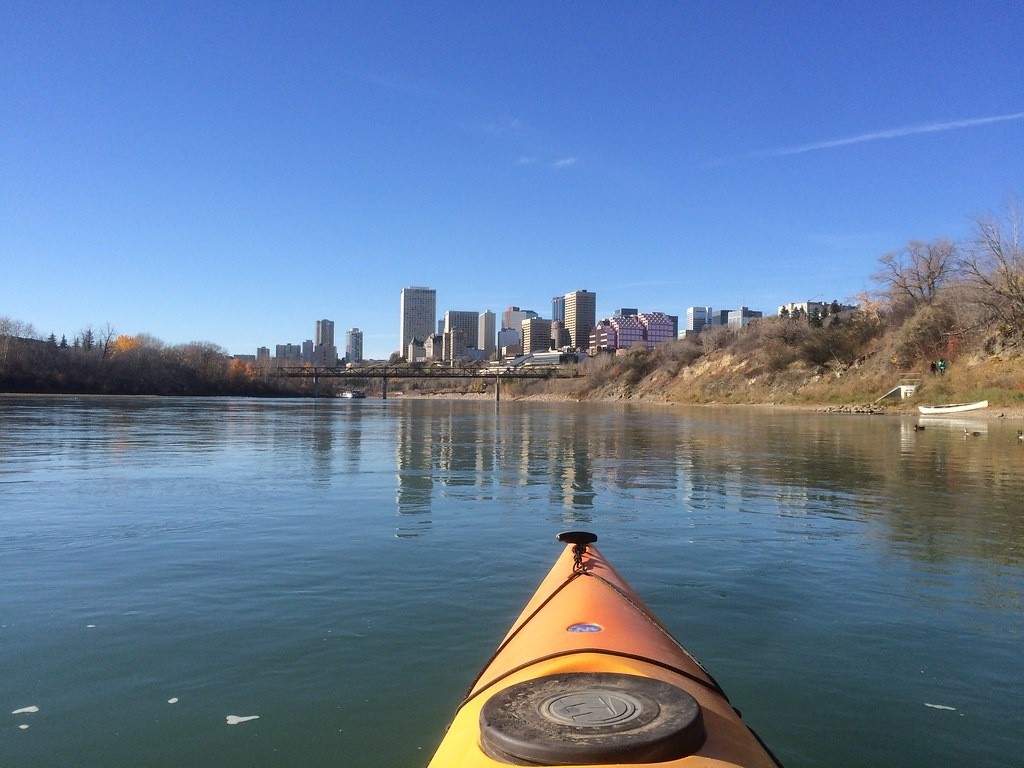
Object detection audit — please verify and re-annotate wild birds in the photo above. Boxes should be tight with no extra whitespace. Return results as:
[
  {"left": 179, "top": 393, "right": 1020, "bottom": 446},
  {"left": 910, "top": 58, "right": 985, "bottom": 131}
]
[
  {"left": 963, "top": 428, "right": 980, "bottom": 436},
  {"left": 1016, "top": 430, "right": 1024, "bottom": 439},
  {"left": 913, "top": 424, "right": 925, "bottom": 430}
]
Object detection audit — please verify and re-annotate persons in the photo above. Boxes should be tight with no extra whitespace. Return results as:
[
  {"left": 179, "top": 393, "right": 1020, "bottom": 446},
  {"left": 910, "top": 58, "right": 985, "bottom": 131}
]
[
  {"left": 930, "top": 361, "right": 935, "bottom": 374},
  {"left": 939, "top": 358, "right": 946, "bottom": 376}
]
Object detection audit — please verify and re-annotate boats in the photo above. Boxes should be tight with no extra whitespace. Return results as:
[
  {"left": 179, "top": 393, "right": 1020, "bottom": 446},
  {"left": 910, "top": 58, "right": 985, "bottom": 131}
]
[
  {"left": 427, "top": 531, "right": 784, "bottom": 768},
  {"left": 918, "top": 399, "right": 989, "bottom": 414},
  {"left": 346, "top": 390, "right": 366, "bottom": 398}
]
